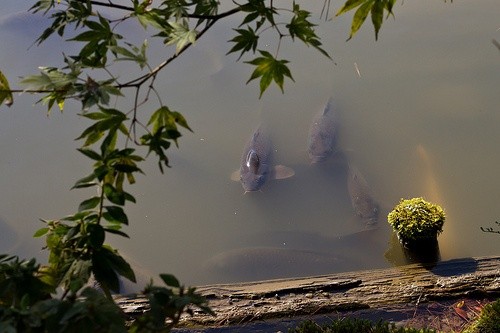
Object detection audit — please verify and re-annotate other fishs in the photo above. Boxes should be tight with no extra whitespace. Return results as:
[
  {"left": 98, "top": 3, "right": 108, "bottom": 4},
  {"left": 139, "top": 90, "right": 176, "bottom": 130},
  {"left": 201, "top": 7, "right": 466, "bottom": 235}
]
[
  {"left": 345, "top": 138, "right": 384, "bottom": 233},
  {"left": 308, "top": 80, "right": 345, "bottom": 165},
  {"left": 230, "top": 122, "right": 297, "bottom": 197},
  {"left": 0, "top": 218, "right": 155, "bottom": 297},
  {"left": 196, "top": 246, "right": 373, "bottom": 277},
  {"left": 414, "top": 144, "right": 458, "bottom": 262},
  {"left": 1, "top": 9, "right": 147, "bottom": 35}
]
